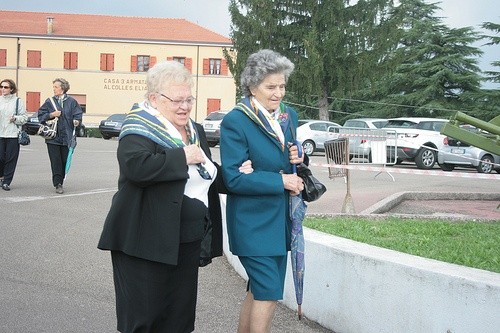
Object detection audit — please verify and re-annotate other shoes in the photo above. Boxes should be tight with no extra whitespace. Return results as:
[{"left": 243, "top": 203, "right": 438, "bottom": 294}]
[
  {"left": 157, "top": 91, "right": 196, "bottom": 106},
  {"left": 2, "top": 183, "right": 10, "bottom": 190},
  {"left": 55, "top": 184, "right": 64, "bottom": 194},
  {"left": 0, "top": 177, "right": 4, "bottom": 187}
]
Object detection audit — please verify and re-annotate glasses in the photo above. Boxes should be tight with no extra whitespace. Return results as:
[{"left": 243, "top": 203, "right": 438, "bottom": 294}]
[{"left": 0, "top": 86, "right": 10, "bottom": 89}]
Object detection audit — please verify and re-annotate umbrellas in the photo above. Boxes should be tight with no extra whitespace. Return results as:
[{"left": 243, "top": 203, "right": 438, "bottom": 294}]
[
  {"left": 63, "top": 120, "right": 77, "bottom": 181},
  {"left": 289, "top": 140, "right": 307, "bottom": 321}
]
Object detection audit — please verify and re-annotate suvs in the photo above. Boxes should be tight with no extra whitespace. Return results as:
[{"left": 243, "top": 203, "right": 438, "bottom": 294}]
[
  {"left": 381, "top": 117, "right": 455, "bottom": 170},
  {"left": 200, "top": 111, "right": 230, "bottom": 148}
]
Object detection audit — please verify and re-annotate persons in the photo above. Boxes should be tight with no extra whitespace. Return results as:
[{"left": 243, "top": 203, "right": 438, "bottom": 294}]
[
  {"left": 0, "top": 79, "right": 29, "bottom": 191},
  {"left": 96, "top": 60, "right": 254, "bottom": 333},
  {"left": 220, "top": 49, "right": 309, "bottom": 333},
  {"left": 38, "top": 78, "right": 83, "bottom": 195}
]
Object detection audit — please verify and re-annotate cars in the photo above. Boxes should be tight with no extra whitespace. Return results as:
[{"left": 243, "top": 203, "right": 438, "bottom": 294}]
[
  {"left": 99, "top": 113, "right": 129, "bottom": 140},
  {"left": 22, "top": 112, "right": 87, "bottom": 138},
  {"left": 295, "top": 119, "right": 343, "bottom": 156},
  {"left": 437, "top": 124, "right": 500, "bottom": 174}
]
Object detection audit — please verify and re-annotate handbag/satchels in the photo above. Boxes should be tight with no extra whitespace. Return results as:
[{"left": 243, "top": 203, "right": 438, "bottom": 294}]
[
  {"left": 37, "top": 97, "right": 59, "bottom": 139},
  {"left": 18, "top": 130, "right": 30, "bottom": 145},
  {"left": 285, "top": 106, "right": 327, "bottom": 203}
]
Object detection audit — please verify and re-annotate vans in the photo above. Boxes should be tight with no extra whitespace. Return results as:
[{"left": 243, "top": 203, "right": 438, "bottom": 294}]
[{"left": 338, "top": 118, "right": 388, "bottom": 165}]
